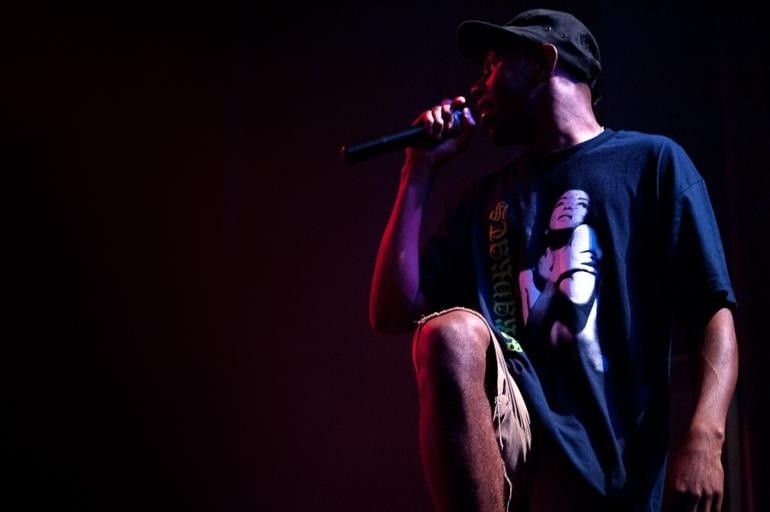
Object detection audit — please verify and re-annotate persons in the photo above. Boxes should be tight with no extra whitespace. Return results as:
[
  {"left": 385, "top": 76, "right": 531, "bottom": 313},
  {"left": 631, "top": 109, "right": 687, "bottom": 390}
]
[
  {"left": 517, "top": 188, "right": 607, "bottom": 372},
  {"left": 368, "top": 9, "right": 739, "bottom": 512}
]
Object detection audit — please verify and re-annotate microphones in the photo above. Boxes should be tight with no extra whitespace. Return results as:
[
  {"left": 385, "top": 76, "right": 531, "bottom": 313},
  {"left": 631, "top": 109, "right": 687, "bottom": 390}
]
[{"left": 344, "top": 106, "right": 474, "bottom": 163}]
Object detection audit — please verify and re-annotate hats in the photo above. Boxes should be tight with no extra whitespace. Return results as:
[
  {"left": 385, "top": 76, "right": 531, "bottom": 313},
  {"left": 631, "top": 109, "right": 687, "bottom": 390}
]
[{"left": 456, "top": 9, "right": 602, "bottom": 88}]
